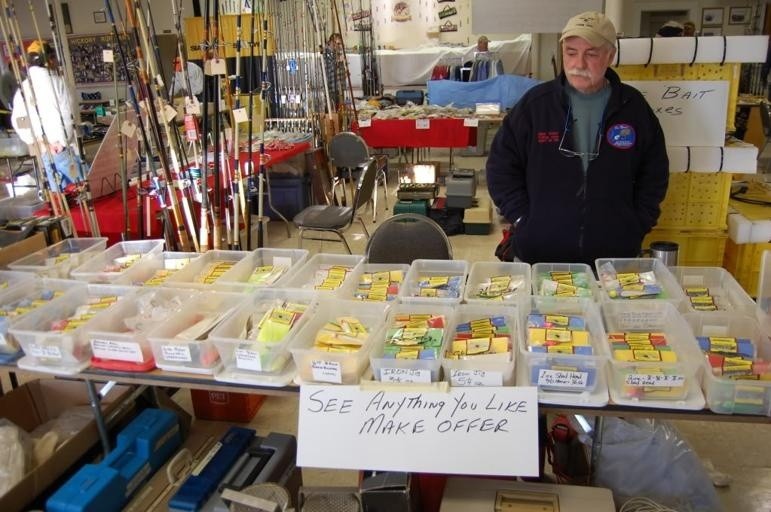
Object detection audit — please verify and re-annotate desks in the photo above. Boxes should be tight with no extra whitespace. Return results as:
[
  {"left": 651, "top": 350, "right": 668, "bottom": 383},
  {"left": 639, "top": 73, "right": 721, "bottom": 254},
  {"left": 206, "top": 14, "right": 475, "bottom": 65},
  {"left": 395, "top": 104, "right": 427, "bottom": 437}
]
[
  {"left": 351, "top": 105, "right": 481, "bottom": 176},
  {"left": 0, "top": 137, "right": 40, "bottom": 197},
  {"left": 39, "top": 138, "right": 311, "bottom": 248},
  {"left": 78, "top": 97, "right": 111, "bottom": 126},
  {"left": 459, "top": 112, "right": 506, "bottom": 158}
]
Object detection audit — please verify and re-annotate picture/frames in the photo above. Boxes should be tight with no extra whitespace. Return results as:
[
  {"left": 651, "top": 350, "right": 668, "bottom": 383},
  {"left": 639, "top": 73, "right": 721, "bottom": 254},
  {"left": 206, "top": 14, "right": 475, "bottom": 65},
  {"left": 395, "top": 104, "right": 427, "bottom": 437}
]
[
  {"left": 700, "top": 5, "right": 753, "bottom": 37},
  {"left": 48, "top": 3, "right": 75, "bottom": 35},
  {"left": 93, "top": 11, "right": 107, "bottom": 24}
]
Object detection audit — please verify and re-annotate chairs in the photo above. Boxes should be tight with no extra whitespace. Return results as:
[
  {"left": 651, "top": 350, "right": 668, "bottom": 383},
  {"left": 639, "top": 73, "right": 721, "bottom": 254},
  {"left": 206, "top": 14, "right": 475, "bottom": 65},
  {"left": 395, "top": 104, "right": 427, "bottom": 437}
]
[
  {"left": 363, "top": 211, "right": 453, "bottom": 266},
  {"left": 326, "top": 130, "right": 391, "bottom": 224},
  {"left": 292, "top": 154, "right": 381, "bottom": 255},
  {"left": 394, "top": 89, "right": 425, "bottom": 165}
]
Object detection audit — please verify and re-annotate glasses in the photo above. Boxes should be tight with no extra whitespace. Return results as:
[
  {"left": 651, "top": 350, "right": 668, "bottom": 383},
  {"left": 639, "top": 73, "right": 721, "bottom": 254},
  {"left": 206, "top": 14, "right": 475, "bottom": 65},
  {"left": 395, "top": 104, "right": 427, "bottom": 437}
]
[{"left": 558, "top": 128, "right": 602, "bottom": 161}]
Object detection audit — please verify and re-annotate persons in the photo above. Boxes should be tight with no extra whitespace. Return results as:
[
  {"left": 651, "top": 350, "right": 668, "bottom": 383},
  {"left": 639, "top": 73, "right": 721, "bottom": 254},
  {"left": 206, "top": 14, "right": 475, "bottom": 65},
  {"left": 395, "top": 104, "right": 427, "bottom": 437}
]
[
  {"left": 168, "top": 57, "right": 204, "bottom": 102},
  {"left": 0, "top": 59, "right": 27, "bottom": 111},
  {"left": 11, "top": 40, "right": 92, "bottom": 193},
  {"left": 486, "top": 12, "right": 668, "bottom": 271}
]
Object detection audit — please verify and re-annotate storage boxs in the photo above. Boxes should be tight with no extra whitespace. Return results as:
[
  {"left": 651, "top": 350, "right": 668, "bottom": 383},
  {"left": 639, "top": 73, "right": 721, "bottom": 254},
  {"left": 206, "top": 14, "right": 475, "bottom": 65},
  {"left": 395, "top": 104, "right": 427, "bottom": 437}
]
[
  {"left": 438, "top": 475, "right": 617, "bottom": 512},
  {"left": 367, "top": 258, "right": 771, "bottom": 415},
  {"left": 0, "top": 227, "right": 419, "bottom": 512},
  {"left": 392, "top": 167, "right": 494, "bottom": 237}
]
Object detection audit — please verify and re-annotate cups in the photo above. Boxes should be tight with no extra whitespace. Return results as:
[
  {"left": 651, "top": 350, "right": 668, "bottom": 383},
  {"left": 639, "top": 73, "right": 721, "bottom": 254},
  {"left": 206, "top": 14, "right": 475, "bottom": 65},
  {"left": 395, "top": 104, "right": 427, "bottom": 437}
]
[{"left": 638, "top": 240, "right": 679, "bottom": 266}]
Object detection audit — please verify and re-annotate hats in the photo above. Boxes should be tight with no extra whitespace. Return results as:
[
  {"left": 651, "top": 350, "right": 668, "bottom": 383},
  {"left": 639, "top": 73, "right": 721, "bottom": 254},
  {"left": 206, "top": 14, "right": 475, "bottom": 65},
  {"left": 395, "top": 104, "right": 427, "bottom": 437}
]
[
  {"left": 26, "top": 39, "right": 56, "bottom": 60},
  {"left": 477, "top": 36, "right": 491, "bottom": 43},
  {"left": 558, "top": 11, "right": 617, "bottom": 48}
]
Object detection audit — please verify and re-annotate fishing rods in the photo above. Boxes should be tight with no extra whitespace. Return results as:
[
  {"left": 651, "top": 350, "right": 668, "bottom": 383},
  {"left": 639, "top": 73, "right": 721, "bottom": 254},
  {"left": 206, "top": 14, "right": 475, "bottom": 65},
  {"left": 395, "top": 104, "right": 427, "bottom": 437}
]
[
  {"left": 268, "top": 1, "right": 382, "bottom": 222},
  {"left": 0, "top": 1, "right": 102, "bottom": 240},
  {"left": 106, "top": 1, "right": 268, "bottom": 252}
]
